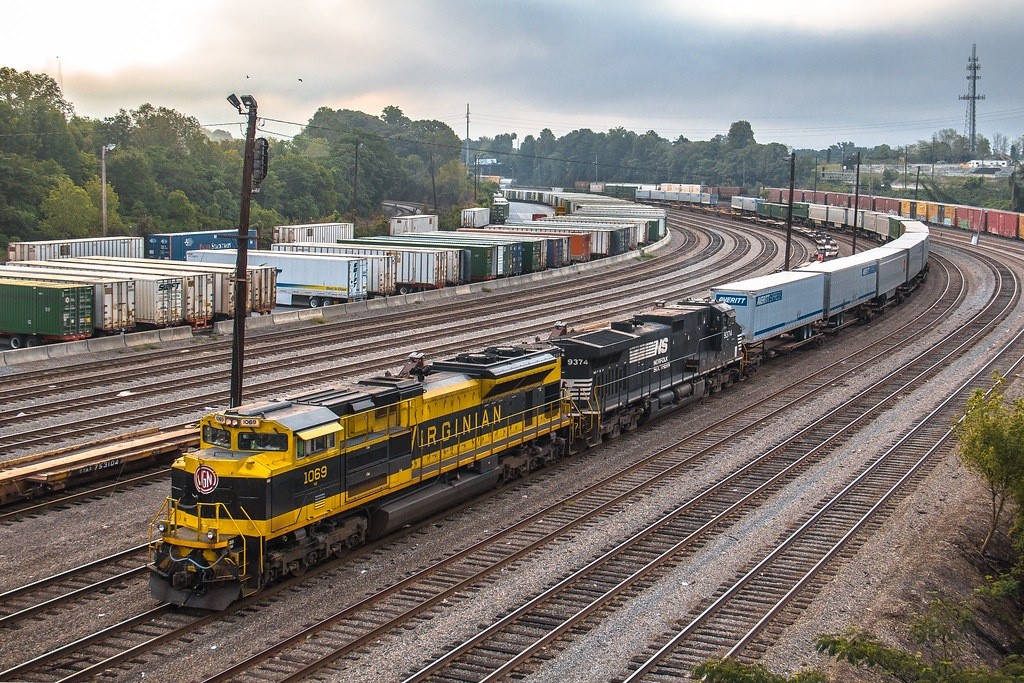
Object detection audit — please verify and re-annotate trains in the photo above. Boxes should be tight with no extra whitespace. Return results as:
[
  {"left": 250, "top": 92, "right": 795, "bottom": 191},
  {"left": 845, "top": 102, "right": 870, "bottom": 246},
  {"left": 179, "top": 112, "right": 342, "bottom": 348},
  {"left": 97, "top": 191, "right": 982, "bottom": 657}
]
[
  {"left": 145, "top": 192, "right": 931, "bottom": 617},
  {"left": 573, "top": 181, "right": 1023, "bottom": 240}
]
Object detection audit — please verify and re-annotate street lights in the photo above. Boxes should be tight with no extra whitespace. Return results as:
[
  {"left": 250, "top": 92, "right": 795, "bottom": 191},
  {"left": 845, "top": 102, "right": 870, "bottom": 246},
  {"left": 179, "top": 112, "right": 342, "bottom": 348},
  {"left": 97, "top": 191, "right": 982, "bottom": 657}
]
[{"left": 100, "top": 143, "right": 117, "bottom": 234}]
